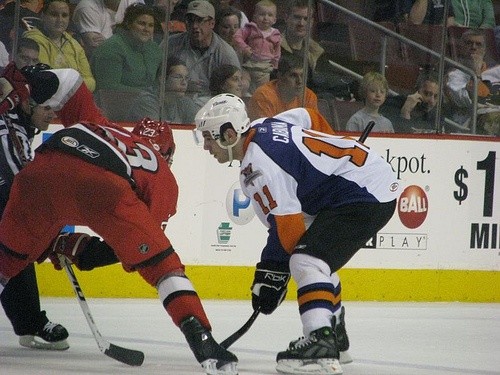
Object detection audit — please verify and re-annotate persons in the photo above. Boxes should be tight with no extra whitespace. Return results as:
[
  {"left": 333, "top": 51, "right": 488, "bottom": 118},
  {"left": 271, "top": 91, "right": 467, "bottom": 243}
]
[
  {"left": 0, "top": 0, "right": 50, "bottom": 51},
  {"left": 125, "top": 56, "right": 202, "bottom": 124},
  {"left": 213, "top": 6, "right": 242, "bottom": 45},
  {"left": 9, "top": 38, "right": 40, "bottom": 69},
  {"left": 0, "top": 63, "right": 70, "bottom": 350},
  {"left": 451, "top": 0, "right": 496, "bottom": 33},
  {"left": 401, "top": 0, "right": 459, "bottom": 26},
  {"left": 387, "top": 74, "right": 450, "bottom": 133},
  {"left": 443, "top": 29, "right": 500, "bottom": 136},
  {"left": 195, "top": 64, "right": 244, "bottom": 107},
  {"left": 346, "top": 71, "right": 394, "bottom": 133},
  {"left": 232, "top": 0, "right": 281, "bottom": 95},
  {"left": 159, "top": 0, "right": 241, "bottom": 97},
  {"left": 192, "top": 93, "right": 404, "bottom": 375},
  {"left": 246, "top": 53, "right": 317, "bottom": 122},
  {"left": 270, "top": 0, "right": 324, "bottom": 84},
  {"left": 71, "top": 0, "right": 113, "bottom": 61},
  {"left": 19, "top": 0, "right": 96, "bottom": 93},
  {"left": 89, "top": 2, "right": 163, "bottom": 123},
  {"left": 0, "top": 41, "right": 239, "bottom": 375}
]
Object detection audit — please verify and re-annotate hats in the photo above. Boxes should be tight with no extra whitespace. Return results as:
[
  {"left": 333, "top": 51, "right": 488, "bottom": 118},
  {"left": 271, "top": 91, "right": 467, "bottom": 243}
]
[{"left": 183, "top": 1, "right": 215, "bottom": 20}]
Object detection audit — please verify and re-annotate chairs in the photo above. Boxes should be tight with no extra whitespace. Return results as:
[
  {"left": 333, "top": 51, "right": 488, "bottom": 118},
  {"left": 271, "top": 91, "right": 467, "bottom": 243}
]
[{"left": 241, "top": 0, "right": 500, "bottom": 134}]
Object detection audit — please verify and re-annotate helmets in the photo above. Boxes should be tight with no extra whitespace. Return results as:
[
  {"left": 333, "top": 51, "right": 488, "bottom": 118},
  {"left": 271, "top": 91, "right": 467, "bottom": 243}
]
[
  {"left": 20, "top": 63, "right": 53, "bottom": 81},
  {"left": 132, "top": 116, "right": 176, "bottom": 154},
  {"left": 195, "top": 93, "right": 250, "bottom": 134}
]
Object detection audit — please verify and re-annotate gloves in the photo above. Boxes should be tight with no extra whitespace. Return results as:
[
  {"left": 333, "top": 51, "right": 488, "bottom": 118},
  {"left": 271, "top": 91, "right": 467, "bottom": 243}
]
[
  {"left": 37, "top": 232, "right": 101, "bottom": 271},
  {"left": 0, "top": 62, "right": 32, "bottom": 117},
  {"left": 249, "top": 260, "right": 290, "bottom": 316}
]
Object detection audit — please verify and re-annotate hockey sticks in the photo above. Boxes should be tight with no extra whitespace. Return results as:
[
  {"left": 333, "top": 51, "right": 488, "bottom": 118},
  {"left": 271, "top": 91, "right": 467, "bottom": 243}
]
[
  {"left": 217, "top": 121, "right": 375, "bottom": 345},
  {"left": 3, "top": 109, "right": 145, "bottom": 366}
]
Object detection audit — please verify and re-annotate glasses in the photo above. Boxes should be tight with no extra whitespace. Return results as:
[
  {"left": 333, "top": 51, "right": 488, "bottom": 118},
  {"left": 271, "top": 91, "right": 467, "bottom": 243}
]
[
  {"left": 167, "top": 75, "right": 191, "bottom": 82},
  {"left": 185, "top": 16, "right": 210, "bottom": 24}
]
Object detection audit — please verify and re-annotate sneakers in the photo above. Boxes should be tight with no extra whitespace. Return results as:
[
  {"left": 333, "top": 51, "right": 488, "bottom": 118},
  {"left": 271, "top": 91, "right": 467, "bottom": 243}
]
[
  {"left": 180, "top": 316, "right": 238, "bottom": 375},
  {"left": 13, "top": 310, "right": 69, "bottom": 350},
  {"left": 275, "top": 330, "right": 342, "bottom": 374},
  {"left": 288, "top": 322, "right": 353, "bottom": 364}
]
